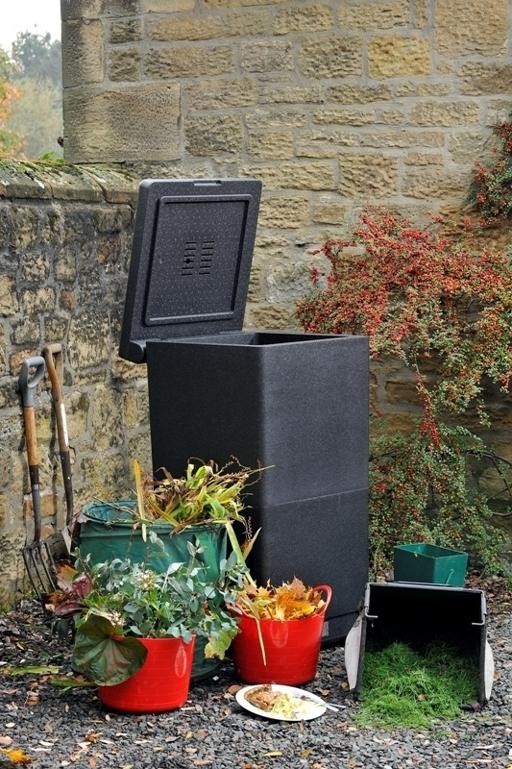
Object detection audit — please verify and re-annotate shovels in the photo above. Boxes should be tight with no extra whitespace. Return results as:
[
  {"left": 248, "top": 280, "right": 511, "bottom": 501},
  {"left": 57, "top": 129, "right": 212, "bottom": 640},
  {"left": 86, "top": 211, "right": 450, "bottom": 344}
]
[{"left": 44, "top": 343, "right": 87, "bottom": 557}]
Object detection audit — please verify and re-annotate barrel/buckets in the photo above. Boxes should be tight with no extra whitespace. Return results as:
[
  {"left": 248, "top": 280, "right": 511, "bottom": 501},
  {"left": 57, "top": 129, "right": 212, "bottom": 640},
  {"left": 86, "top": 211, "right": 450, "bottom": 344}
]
[
  {"left": 223, "top": 584, "right": 332, "bottom": 684},
  {"left": 76, "top": 500, "right": 231, "bottom": 679},
  {"left": 95, "top": 632, "right": 195, "bottom": 713}
]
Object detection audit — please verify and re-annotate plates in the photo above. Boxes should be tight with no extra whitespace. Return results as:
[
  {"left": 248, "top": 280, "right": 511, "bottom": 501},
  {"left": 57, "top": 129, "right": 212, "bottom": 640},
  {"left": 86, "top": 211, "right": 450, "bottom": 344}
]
[{"left": 235, "top": 684, "right": 328, "bottom": 723}]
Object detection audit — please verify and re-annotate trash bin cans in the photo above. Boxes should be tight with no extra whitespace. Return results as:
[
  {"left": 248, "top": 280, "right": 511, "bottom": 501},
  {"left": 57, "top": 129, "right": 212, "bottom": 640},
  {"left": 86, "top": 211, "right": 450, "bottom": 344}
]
[
  {"left": 119, "top": 178, "right": 469, "bottom": 679},
  {"left": 76, "top": 502, "right": 228, "bottom": 603},
  {"left": 392, "top": 545, "right": 469, "bottom": 591}
]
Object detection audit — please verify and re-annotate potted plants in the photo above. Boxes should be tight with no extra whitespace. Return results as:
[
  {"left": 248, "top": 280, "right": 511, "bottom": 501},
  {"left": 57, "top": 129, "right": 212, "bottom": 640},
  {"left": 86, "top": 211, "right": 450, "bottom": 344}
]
[{"left": 57, "top": 559, "right": 236, "bottom": 716}]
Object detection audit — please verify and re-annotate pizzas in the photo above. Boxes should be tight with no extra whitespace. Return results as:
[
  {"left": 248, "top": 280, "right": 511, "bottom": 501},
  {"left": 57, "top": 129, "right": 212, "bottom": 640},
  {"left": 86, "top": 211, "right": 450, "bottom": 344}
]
[{"left": 243, "top": 684, "right": 275, "bottom": 710}]
